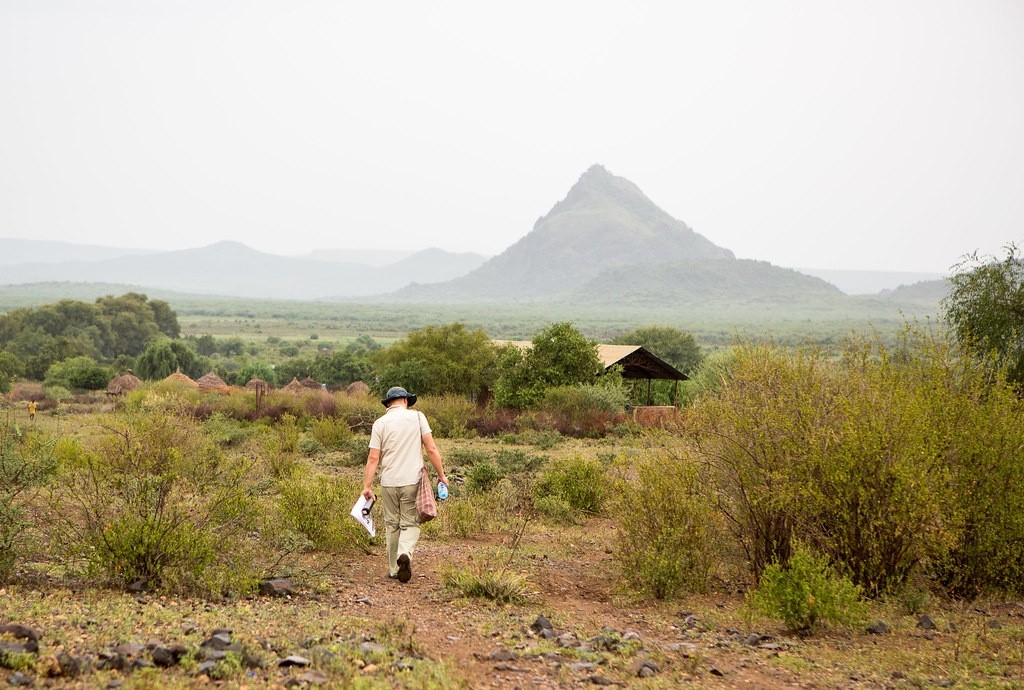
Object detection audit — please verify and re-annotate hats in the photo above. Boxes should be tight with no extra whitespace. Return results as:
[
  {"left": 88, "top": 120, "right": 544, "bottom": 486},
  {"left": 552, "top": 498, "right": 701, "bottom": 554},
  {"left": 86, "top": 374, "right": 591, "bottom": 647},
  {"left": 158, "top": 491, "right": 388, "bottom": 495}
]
[{"left": 381, "top": 387, "right": 417, "bottom": 408}]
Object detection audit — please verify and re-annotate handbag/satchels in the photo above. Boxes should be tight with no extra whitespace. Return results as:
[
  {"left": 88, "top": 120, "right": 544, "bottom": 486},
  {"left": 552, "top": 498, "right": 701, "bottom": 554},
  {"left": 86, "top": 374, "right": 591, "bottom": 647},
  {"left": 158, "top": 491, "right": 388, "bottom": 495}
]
[{"left": 415, "top": 466, "right": 437, "bottom": 523}]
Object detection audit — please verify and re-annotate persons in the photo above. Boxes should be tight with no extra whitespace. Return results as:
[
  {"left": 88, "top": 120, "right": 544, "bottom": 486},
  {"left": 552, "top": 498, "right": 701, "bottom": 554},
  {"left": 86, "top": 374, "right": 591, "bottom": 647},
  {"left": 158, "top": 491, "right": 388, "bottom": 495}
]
[{"left": 362, "top": 386, "right": 448, "bottom": 583}]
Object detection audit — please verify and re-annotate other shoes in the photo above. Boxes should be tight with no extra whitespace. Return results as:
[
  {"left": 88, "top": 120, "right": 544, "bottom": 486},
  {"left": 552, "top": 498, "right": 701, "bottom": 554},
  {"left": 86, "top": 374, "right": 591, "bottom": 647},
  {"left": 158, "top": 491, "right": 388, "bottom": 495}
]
[
  {"left": 389, "top": 574, "right": 398, "bottom": 578},
  {"left": 397, "top": 554, "right": 411, "bottom": 583}
]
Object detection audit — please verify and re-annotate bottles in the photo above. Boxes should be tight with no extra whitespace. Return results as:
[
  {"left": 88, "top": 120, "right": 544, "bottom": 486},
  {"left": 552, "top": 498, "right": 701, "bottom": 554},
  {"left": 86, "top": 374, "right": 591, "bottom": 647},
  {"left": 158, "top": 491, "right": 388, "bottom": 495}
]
[{"left": 437, "top": 482, "right": 448, "bottom": 499}]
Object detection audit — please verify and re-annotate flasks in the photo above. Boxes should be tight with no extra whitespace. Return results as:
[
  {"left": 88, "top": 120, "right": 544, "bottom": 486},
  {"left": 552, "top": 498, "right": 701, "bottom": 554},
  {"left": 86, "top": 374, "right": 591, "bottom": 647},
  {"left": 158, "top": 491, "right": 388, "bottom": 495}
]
[{"left": 361, "top": 495, "right": 377, "bottom": 515}]
